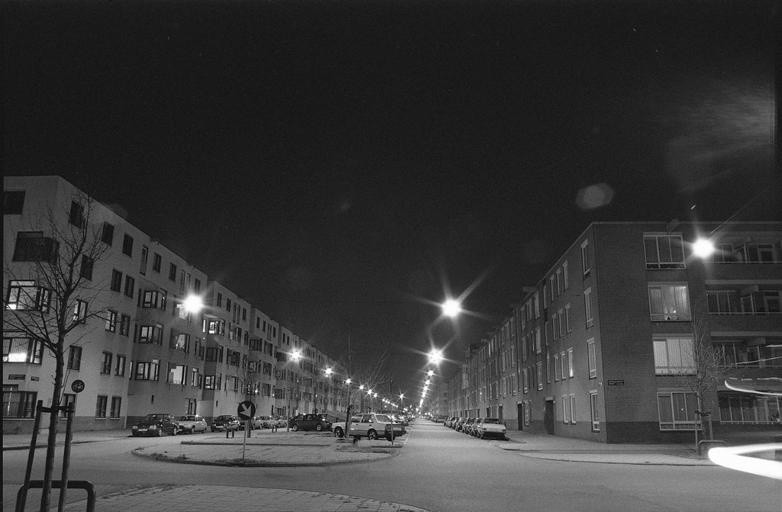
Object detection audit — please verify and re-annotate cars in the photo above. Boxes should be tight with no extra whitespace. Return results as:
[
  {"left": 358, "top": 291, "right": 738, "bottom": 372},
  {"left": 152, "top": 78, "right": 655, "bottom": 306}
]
[
  {"left": 331, "top": 409, "right": 416, "bottom": 442},
  {"left": 130, "top": 411, "right": 346, "bottom": 440},
  {"left": 420, "top": 412, "right": 508, "bottom": 441}
]
